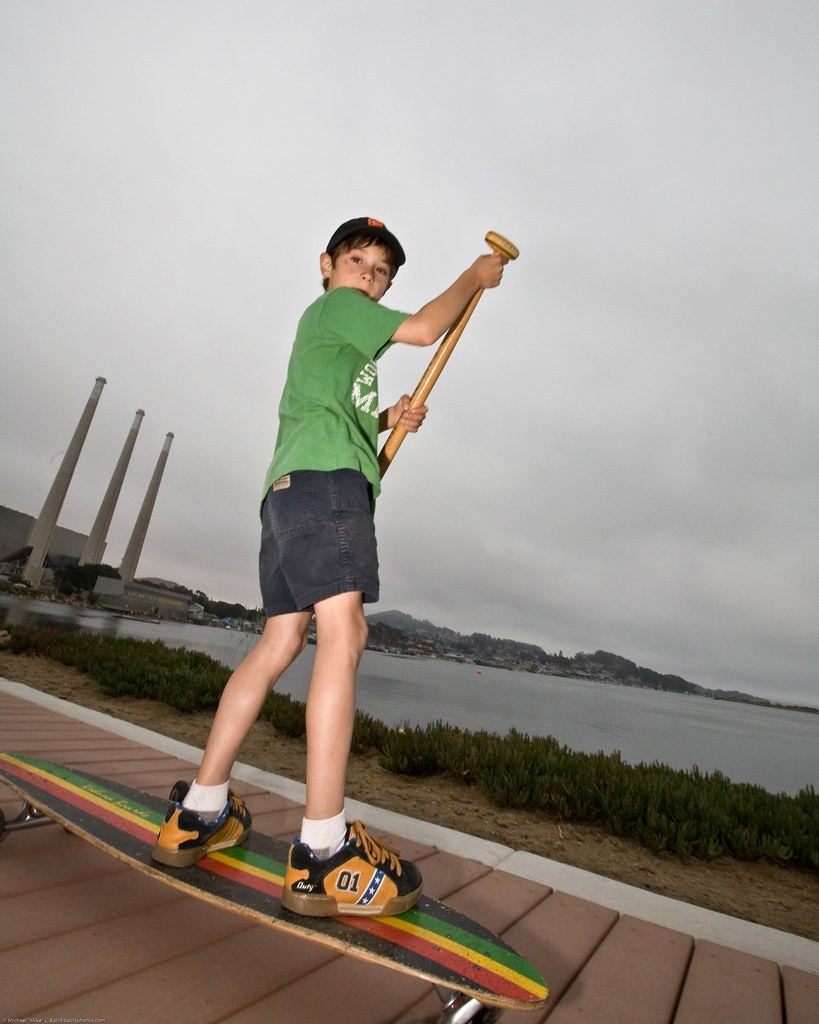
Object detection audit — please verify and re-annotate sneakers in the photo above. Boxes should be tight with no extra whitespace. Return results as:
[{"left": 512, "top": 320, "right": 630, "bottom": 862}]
[
  {"left": 152, "top": 780, "right": 252, "bottom": 868},
  {"left": 280, "top": 819, "right": 423, "bottom": 917}
]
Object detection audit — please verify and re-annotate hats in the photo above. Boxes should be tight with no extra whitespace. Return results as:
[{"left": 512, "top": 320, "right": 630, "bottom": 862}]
[{"left": 326, "top": 217, "right": 406, "bottom": 266}]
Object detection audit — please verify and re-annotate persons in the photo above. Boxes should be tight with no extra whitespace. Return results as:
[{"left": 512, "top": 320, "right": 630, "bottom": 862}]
[{"left": 148, "top": 216, "right": 510, "bottom": 917}]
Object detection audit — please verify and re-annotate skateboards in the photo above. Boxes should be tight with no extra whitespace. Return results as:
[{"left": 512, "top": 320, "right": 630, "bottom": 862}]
[{"left": 0, "top": 748, "right": 550, "bottom": 1024}]
